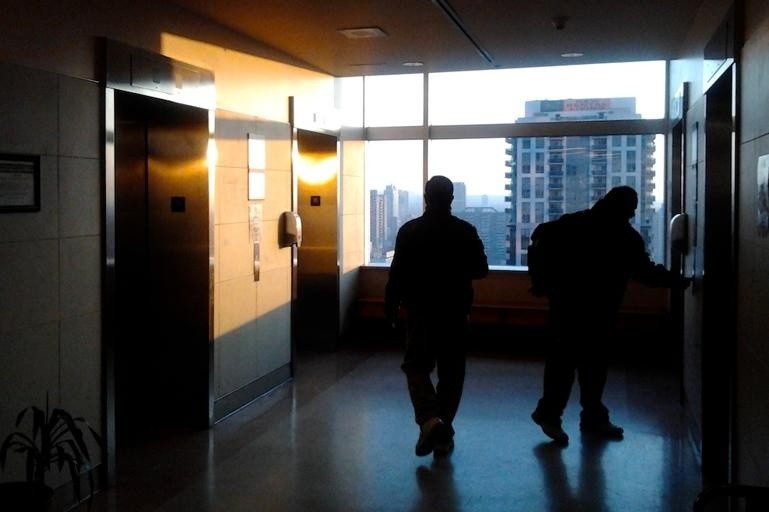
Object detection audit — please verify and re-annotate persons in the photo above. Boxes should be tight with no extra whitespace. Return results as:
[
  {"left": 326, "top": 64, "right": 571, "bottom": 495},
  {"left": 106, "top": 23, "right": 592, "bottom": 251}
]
[
  {"left": 530, "top": 185, "right": 693, "bottom": 444},
  {"left": 383, "top": 175, "right": 490, "bottom": 459}
]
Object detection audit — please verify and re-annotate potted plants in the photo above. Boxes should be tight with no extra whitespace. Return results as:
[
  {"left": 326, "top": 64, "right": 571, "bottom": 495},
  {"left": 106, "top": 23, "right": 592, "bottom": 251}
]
[{"left": 0, "top": 391, "right": 106, "bottom": 512}]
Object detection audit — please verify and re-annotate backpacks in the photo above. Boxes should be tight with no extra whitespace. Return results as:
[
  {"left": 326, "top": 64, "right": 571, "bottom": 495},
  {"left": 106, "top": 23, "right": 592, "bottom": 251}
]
[{"left": 527, "top": 219, "right": 574, "bottom": 297}]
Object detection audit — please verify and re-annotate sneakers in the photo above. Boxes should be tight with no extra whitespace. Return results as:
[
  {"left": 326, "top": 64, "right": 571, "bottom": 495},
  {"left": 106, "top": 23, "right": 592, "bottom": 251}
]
[
  {"left": 581, "top": 420, "right": 623, "bottom": 435},
  {"left": 416, "top": 417, "right": 454, "bottom": 457},
  {"left": 531, "top": 409, "right": 568, "bottom": 442}
]
